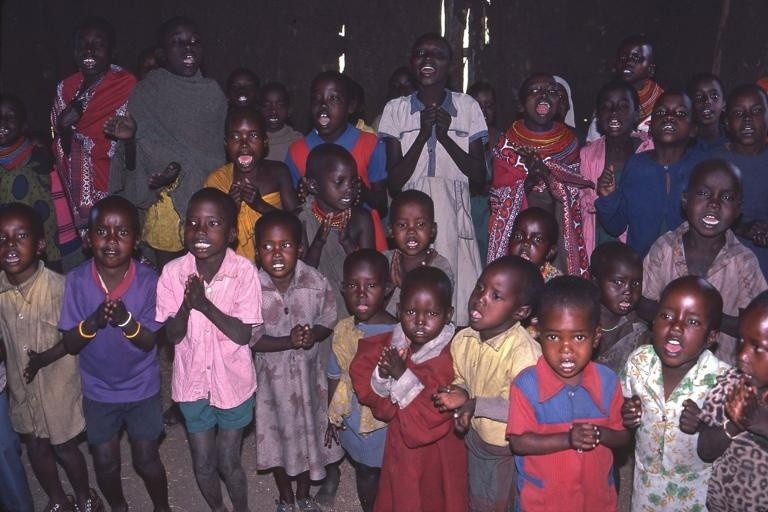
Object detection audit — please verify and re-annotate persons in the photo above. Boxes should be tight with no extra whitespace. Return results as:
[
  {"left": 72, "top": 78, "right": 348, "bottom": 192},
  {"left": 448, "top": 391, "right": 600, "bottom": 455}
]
[{"left": 1, "top": 17, "right": 766, "bottom": 511}]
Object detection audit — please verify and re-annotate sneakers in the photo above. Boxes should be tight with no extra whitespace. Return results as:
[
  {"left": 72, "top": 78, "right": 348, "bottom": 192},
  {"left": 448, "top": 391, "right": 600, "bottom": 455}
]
[
  {"left": 46, "top": 488, "right": 104, "bottom": 512},
  {"left": 278, "top": 497, "right": 322, "bottom": 512}
]
